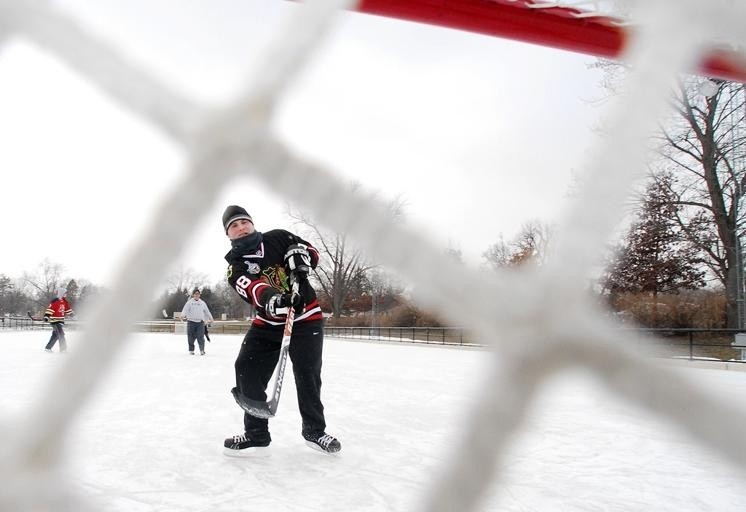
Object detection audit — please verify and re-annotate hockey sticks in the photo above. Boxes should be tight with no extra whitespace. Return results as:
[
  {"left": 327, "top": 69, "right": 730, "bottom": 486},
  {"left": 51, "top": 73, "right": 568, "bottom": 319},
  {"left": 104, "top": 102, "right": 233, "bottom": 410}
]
[
  {"left": 231, "top": 283, "right": 299, "bottom": 419},
  {"left": 162, "top": 310, "right": 185, "bottom": 319},
  {"left": 27, "top": 313, "right": 64, "bottom": 322}
]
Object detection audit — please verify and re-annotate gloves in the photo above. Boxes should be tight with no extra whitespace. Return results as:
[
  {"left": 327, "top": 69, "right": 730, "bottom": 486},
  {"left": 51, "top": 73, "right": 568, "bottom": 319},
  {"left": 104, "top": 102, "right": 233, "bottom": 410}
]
[
  {"left": 283, "top": 244, "right": 313, "bottom": 281},
  {"left": 264, "top": 292, "right": 305, "bottom": 322}
]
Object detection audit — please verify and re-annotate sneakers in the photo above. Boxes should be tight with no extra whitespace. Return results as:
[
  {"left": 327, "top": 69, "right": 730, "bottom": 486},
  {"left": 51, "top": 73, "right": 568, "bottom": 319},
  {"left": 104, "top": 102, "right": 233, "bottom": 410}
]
[
  {"left": 224, "top": 432, "right": 271, "bottom": 450},
  {"left": 301, "top": 430, "right": 342, "bottom": 453}
]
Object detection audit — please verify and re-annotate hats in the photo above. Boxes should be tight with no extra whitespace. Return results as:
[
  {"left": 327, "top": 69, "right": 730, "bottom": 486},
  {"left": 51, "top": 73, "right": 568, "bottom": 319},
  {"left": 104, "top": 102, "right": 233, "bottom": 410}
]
[{"left": 222, "top": 205, "right": 254, "bottom": 235}]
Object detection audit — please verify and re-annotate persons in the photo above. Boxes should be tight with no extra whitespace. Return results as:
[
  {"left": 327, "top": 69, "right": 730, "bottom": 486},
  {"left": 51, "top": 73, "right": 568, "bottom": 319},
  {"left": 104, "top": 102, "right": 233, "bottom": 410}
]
[
  {"left": 182, "top": 289, "right": 214, "bottom": 355},
  {"left": 222, "top": 206, "right": 341, "bottom": 453},
  {"left": 43, "top": 287, "right": 75, "bottom": 353},
  {"left": 193, "top": 320, "right": 211, "bottom": 342}
]
